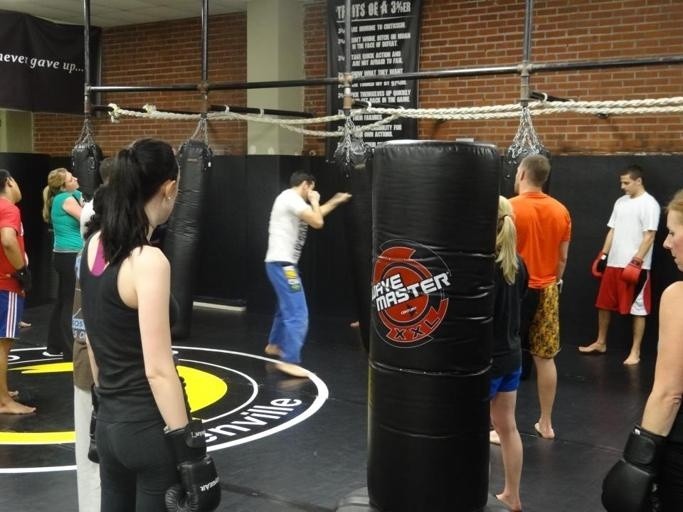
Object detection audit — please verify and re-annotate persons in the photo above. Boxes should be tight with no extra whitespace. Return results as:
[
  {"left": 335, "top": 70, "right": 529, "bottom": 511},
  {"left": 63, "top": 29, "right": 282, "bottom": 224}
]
[
  {"left": 39, "top": 165, "right": 90, "bottom": 364},
  {"left": 71, "top": 183, "right": 112, "bottom": 511},
  {"left": 576, "top": 163, "right": 661, "bottom": 368},
  {"left": 599, "top": 183, "right": 683, "bottom": 512},
  {"left": 77, "top": 137, "right": 223, "bottom": 511},
  {"left": 488, "top": 152, "right": 572, "bottom": 448},
  {"left": 261, "top": 167, "right": 353, "bottom": 380},
  {"left": 0, "top": 165, "right": 38, "bottom": 418},
  {"left": 487, "top": 190, "right": 526, "bottom": 511},
  {"left": 78, "top": 157, "right": 116, "bottom": 240}
]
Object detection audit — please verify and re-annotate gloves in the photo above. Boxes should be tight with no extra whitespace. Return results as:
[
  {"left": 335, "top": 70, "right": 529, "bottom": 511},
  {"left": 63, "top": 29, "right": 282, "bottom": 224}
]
[
  {"left": 12, "top": 265, "right": 37, "bottom": 295},
  {"left": 159, "top": 419, "right": 223, "bottom": 512},
  {"left": 619, "top": 256, "right": 644, "bottom": 283},
  {"left": 590, "top": 250, "right": 608, "bottom": 278},
  {"left": 85, "top": 381, "right": 102, "bottom": 465},
  {"left": 598, "top": 424, "right": 667, "bottom": 512}
]
